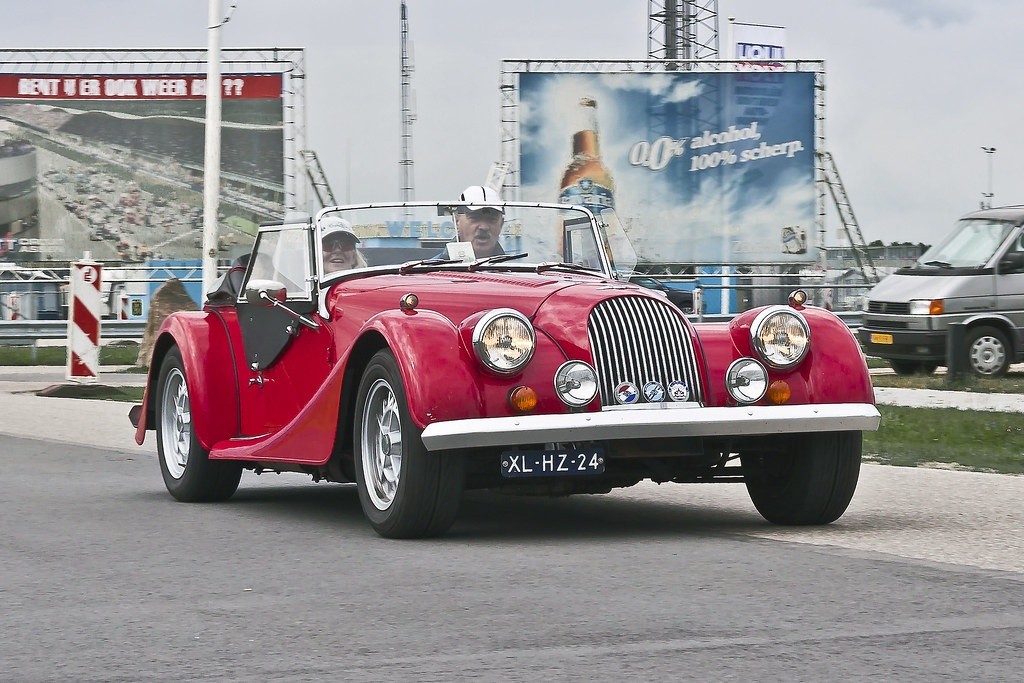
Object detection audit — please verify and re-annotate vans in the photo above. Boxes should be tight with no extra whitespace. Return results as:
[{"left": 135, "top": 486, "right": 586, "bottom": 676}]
[{"left": 856, "top": 204, "right": 1024, "bottom": 380}]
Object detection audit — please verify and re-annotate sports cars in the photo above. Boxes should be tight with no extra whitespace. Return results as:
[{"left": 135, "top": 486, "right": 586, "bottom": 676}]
[{"left": 129, "top": 201, "right": 882, "bottom": 542}]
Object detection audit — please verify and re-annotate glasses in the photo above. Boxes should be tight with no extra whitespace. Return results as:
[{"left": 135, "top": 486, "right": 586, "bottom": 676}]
[{"left": 322, "top": 237, "right": 355, "bottom": 252}]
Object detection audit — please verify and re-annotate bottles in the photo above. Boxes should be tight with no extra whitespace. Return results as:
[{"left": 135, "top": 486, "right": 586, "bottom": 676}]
[
  {"left": 555, "top": 95, "right": 618, "bottom": 265},
  {"left": 795, "top": 225, "right": 808, "bottom": 253}
]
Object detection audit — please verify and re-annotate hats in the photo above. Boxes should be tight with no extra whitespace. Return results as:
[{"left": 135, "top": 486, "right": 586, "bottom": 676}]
[
  {"left": 457, "top": 186, "right": 506, "bottom": 215},
  {"left": 320, "top": 216, "right": 361, "bottom": 245}
]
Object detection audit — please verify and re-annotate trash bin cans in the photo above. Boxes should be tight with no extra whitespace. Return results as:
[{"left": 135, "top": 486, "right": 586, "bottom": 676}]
[{"left": 39, "top": 310, "right": 58, "bottom": 319}]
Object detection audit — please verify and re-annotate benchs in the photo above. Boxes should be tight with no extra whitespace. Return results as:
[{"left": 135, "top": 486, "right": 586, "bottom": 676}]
[{"left": 229, "top": 247, "right": 445, "bottom": 297}]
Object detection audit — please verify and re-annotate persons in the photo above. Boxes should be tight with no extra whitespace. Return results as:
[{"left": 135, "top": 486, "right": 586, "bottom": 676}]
[
  {"left": 432, "top": 186, "right": 505, "bottom": 260},
  {"left": 320, "top": 217, "right": 367, "bottom": 275}
]
